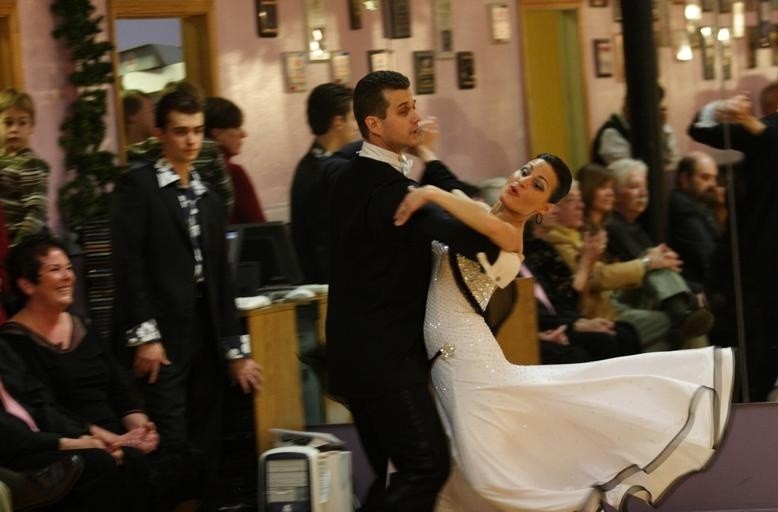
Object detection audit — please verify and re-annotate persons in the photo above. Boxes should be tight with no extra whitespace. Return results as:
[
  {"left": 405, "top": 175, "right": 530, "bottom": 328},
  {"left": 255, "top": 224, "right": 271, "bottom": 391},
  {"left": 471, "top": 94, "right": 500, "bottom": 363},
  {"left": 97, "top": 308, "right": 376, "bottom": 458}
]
[
  {"left": 1, "top": 81, "right": 269, "bottom": 511},
  {"left": 290, "top": 81, "right": 438, "bottom": 285},
  {"left": 321, "top": 72, "right": 525, "bottom": 512},
  {"left": 481, "top": 71, "right": 778, "bottom": 409},
  {"left": 395, "top": 116, "right": 735, "bottom": 512}
]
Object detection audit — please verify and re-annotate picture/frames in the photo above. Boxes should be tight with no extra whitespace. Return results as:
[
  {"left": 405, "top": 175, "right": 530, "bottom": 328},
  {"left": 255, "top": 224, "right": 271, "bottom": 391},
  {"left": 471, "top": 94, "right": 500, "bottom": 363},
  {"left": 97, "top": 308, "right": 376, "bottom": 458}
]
[{"left": 253, "top": 0, "right": 475, "bottom": 95}]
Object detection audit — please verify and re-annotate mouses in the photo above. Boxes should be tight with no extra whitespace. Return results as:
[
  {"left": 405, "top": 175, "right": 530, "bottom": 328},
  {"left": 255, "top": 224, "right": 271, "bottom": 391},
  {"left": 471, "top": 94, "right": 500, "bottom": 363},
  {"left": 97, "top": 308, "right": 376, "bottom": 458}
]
[{"left": 285, "top": 288, "right": 315, "bottom": 300}]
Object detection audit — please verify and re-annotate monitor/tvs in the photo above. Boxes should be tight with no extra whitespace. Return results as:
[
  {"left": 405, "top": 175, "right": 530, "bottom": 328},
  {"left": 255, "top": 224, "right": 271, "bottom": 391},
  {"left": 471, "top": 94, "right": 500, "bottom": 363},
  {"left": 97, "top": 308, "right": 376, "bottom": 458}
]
[
  {"left": 240, "top": 221, "right": 304, "bottom": 297},
  {"left": 224, "top": 226, "right": 245, "bottom": 276}
]
